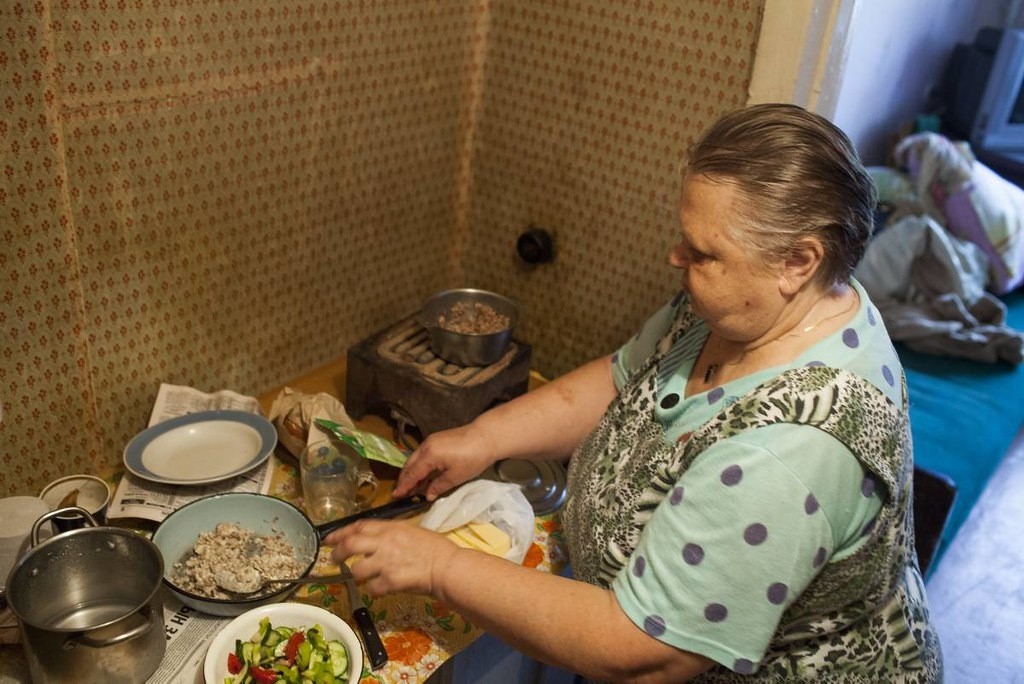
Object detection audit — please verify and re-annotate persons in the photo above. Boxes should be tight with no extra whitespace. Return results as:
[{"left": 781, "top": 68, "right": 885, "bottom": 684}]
[{"left": 324, "top": 104, "right": 944, "bottom": 683}]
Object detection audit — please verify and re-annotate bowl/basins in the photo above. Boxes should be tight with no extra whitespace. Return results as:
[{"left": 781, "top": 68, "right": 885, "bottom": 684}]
[{"left": 202, "top": 600, "right": 364, "bottom": 684}]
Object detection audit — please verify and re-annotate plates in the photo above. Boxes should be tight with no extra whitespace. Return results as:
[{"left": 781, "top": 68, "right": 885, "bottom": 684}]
[{"left": 123, "top": 409, "right": 278, "bottom": 484}]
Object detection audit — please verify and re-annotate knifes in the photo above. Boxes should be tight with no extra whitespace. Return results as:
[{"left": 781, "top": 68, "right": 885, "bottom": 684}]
[{"left": 340, "top": 561, "right": 388, "bottom": 670}]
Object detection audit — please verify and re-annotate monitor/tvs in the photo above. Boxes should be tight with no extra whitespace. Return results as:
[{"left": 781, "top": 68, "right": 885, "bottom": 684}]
[{"left": 935, "top": 25, "right": 1024, "bottom": 150}]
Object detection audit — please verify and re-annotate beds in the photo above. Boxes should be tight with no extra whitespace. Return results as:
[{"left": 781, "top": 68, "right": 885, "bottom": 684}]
[{"left": 849, "top": 139, "right": 1024, "bottom": 585}]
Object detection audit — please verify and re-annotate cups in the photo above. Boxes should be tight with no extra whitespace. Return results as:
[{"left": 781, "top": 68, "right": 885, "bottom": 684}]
[
  {"left": 300, "top": 439, "right": 380, "bottom": 527},
  {"left": 39, "top": 474, "right": 111, "bottom": 532}
]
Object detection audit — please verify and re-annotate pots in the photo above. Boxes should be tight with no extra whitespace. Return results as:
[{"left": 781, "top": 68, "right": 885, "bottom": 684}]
[
  {"left": 149, "top": 491, "right": 427, "bottom": 616},
  {"left": 416, "top": 286, "right": 521, "bottom": 367},
  {"left": 4, "top": 507, "right": 166, "bottom": 683}
]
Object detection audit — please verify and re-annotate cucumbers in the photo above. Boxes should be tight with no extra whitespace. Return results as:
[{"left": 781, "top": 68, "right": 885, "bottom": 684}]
[{"left": 219, "top": 615, "right": 349, "bottom": 684}]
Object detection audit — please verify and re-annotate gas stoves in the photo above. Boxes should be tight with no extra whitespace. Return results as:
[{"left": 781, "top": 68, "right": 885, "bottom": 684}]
[{"left": 344, "top": 307, "right": 532, "bottom": 443}]
